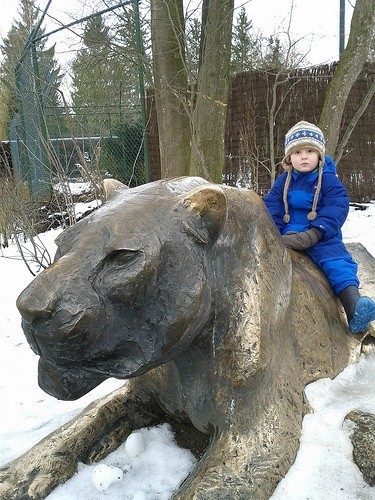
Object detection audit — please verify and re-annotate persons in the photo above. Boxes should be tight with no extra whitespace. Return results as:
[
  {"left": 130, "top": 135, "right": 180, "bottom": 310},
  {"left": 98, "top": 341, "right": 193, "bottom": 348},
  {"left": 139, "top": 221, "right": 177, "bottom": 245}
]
[{"left": 265, "top": 120, "right": 375, "bottom": 333}]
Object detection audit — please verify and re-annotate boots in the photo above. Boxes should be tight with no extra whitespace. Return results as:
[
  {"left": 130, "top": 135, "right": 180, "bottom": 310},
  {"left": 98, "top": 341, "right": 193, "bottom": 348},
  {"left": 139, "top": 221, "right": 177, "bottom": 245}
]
[{"left": 338, "top": 285, "right": 375, "bottom": 334}]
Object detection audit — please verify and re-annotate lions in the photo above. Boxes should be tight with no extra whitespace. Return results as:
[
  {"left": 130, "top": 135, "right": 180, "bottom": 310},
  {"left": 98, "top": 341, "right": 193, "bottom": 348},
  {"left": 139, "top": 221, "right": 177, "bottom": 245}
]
[{"left": 2, "top": 171, "right": 375, "bottom": 500}]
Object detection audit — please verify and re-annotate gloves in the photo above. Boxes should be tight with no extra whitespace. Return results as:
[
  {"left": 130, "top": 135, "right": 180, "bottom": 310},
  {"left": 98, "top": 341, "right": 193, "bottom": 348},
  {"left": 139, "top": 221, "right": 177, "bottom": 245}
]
[{"left": 281, "top": 226, "right": 321, "bottom": 251}]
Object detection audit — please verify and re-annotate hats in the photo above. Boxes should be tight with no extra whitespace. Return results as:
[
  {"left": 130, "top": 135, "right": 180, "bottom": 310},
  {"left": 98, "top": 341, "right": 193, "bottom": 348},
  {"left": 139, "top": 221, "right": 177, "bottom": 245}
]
[{"left": 281, "top": 120, "right": 326, "bottom": 224}]
